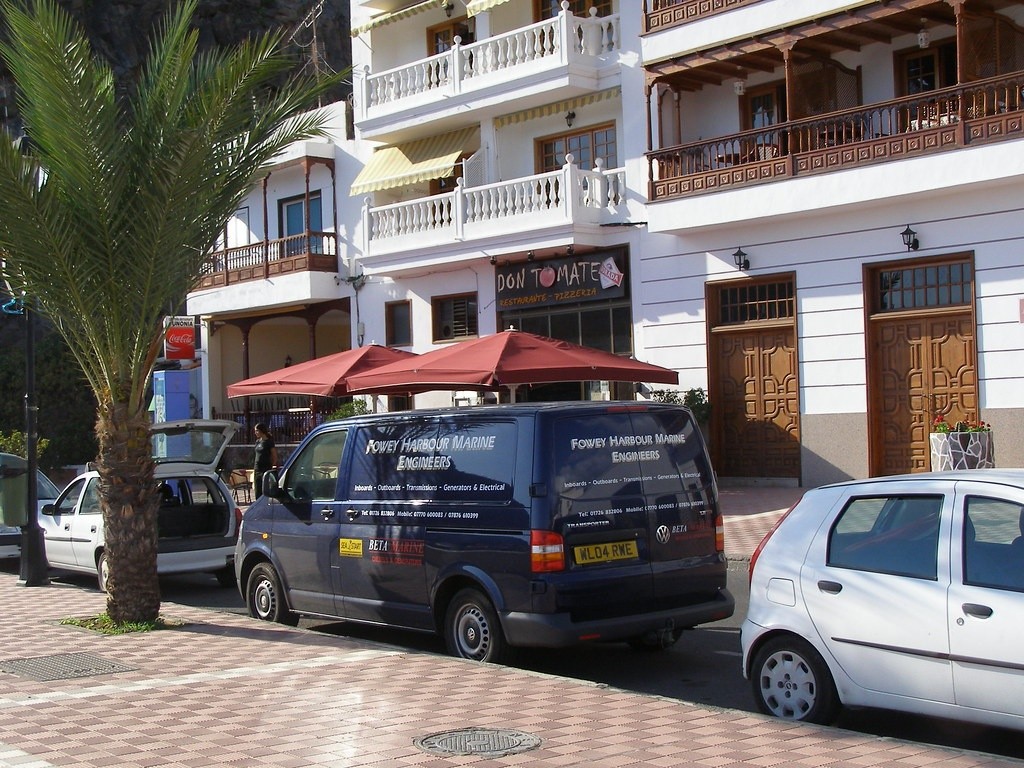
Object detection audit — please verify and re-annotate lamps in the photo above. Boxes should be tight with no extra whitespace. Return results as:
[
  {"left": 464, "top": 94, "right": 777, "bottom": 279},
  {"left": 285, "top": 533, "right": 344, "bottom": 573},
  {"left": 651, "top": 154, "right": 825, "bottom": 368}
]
[
  {"left": 899, "top": 224, "right": 920, "bottom": 252},
  {"left": 490, "top": 256, "right": 498, "bottom": 265},
  {"left": 732, "top": 65, "right": 746, "bottom": 96},
  {"left": 284, "top": 354, "right": 292, "bottom": 368},
  {"left": 565, "top": 111, "right": 576, "bottom": 128},
  {"left": 445, "top": 4, "right": 455, "bottom": 18},
  {"left": 527, "top": 251, "right": 535, "bottom": 262},
  {"left": 565, "top": 245, "right": 574, "bottom": 255},
  {"left": 916, "top": 17, "right": 931, "bottom": 48},
  {"left": 732, "top": 247, "right": 750, "bottom": 271}
]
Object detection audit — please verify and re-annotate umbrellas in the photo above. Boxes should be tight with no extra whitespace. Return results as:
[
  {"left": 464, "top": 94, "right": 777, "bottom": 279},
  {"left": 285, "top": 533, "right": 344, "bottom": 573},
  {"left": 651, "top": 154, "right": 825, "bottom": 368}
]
[
  {"left": 226, "top": 340, "right": 507, "bottom": 413},
  {"left": 345, "top": 324, "right": 679, "bottom": 404}
]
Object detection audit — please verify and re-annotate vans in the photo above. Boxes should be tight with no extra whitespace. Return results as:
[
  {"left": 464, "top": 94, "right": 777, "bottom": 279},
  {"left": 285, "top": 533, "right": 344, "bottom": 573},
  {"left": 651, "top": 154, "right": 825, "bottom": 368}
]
[{"left": 235, "top": 402, "right": 735, "bottom": 662}]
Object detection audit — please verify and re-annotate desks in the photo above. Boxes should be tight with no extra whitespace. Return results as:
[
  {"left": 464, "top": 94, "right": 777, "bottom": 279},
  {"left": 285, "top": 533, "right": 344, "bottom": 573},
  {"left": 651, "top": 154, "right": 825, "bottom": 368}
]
[
  {"left": 714, "top": 152, "right": 747, "bottom": 165},
  {"left": 905, "top": 101, "right": 1006, "bottom": 133},
  {"left": 815, "top": 128, "right": 867, "bottom": 145},
  {"left": 237, "top": 413, "right": 327, "bottom": 442}
]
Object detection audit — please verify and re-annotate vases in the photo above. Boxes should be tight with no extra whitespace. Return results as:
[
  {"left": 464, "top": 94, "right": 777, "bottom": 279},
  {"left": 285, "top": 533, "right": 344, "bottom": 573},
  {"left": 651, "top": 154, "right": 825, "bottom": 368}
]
[
  {"left": 699, "top": 424, "right": 710, "bottom": 444},
  {"left": 929, "top": 432, "right": 996, "bottom": 473}
]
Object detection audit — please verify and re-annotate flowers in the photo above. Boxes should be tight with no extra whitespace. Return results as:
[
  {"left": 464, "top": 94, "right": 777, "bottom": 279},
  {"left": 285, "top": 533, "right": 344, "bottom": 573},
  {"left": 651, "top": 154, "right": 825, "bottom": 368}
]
[
  {"left": 933, "top": 414, "right": 991, "bottom": 433},
  {"left": 651, "top": 386, "right": 713, "bottom": 426}
]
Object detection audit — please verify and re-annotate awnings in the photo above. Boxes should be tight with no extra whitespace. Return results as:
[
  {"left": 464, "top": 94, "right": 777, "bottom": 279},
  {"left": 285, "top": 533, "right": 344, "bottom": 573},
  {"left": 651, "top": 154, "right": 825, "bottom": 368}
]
[
  {"left": 348, "top": 125, "right": 478, "bottom": 198},
  {"left": 467, "top": 0, "right": 511, "bottom": 18}
]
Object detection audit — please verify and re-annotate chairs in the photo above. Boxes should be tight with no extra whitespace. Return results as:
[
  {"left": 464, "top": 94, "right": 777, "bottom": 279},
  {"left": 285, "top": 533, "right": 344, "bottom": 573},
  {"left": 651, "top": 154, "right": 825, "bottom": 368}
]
[
  {"left": 158, "top": 483, "right": 180, "bottom": 505},
  {"left": 206, "top": 468, "right": 253, "bottom": 506},
  {"left": 693, "top": 163, "right": 714, "bottom": 172},
  {"left": 740, "top": 143, "right": 778, "bottom": 164}
]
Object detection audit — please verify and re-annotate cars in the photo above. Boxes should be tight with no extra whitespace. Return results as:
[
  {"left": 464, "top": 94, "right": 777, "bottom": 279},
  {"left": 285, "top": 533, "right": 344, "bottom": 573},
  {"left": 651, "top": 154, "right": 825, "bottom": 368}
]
[
  {"left": 740, "top": 468, "right": 1024, "bottom": 728},
  {"left": 38, "top": 419, "right": 242, "bottom": 595},
  {"left": 0, "top": 468, "right": 72, "bottom": 559}
]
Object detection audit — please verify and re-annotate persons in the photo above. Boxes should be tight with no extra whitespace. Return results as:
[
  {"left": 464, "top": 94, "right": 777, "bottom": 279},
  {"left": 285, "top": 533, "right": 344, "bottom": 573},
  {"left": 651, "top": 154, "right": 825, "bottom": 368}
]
[
  {"left": 251, "top": 423, "right": 278, "bottom": 501},
  {"left": 189, "top": 394, "right": 203, "bottom": 448}
]
